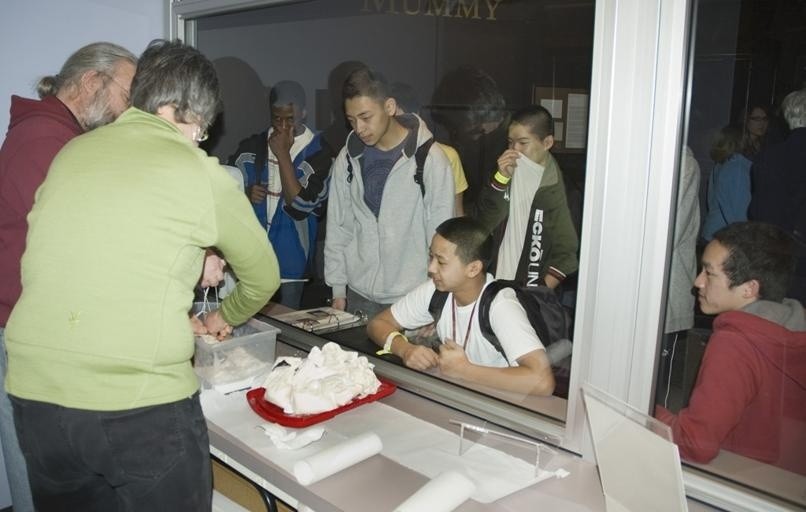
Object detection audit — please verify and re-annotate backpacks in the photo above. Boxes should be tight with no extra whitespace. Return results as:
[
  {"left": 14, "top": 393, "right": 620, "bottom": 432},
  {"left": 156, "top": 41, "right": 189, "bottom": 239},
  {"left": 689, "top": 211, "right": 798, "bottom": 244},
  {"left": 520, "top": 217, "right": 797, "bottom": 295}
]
[{"left": 428, "top": 279, "right": 574, "bottom": 399}]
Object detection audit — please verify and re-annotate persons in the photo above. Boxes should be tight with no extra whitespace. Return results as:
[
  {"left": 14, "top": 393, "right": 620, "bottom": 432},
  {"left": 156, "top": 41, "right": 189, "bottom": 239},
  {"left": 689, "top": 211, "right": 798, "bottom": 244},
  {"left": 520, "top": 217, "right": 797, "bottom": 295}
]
[
  {"left": 3, "top": 37, "right": 281, "bottom": 512},
  {"left": 0, "top": 41, "right": 225, "bottom": 512},
  {"left": 653, "top": 89, "right": 805, "bottom": 473},
  {"left": 229, "top": 70, "right": 580, "bottom": 399}
]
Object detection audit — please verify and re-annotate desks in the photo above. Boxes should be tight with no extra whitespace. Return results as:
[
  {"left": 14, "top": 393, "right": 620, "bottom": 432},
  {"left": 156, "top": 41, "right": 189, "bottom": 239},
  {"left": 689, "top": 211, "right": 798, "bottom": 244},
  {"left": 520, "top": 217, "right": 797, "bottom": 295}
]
[{"left": 199, "top": 368, "right": 726, "bottom": 512}]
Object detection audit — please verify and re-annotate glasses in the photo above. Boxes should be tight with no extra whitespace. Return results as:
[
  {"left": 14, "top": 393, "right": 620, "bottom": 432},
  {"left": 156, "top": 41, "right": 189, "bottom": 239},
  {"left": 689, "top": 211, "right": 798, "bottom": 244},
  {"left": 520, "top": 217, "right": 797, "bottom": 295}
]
[{"left": 747, "top": 115, "right": 768, "bottom": 121}]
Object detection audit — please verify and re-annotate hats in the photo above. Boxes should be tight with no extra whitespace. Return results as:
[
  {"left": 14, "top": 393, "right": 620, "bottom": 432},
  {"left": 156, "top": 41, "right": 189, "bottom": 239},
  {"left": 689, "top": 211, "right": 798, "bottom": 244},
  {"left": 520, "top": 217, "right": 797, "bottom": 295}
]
[{"left": 782, "top": 92, "right": 806, "bottom": 130}]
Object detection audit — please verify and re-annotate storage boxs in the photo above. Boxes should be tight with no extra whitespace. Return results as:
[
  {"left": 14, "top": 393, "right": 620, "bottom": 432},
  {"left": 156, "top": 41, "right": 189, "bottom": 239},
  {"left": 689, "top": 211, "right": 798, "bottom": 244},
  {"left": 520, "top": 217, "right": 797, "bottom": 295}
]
[{"left": 190, "top": 301, "right": 282, "bottom": 387}]
